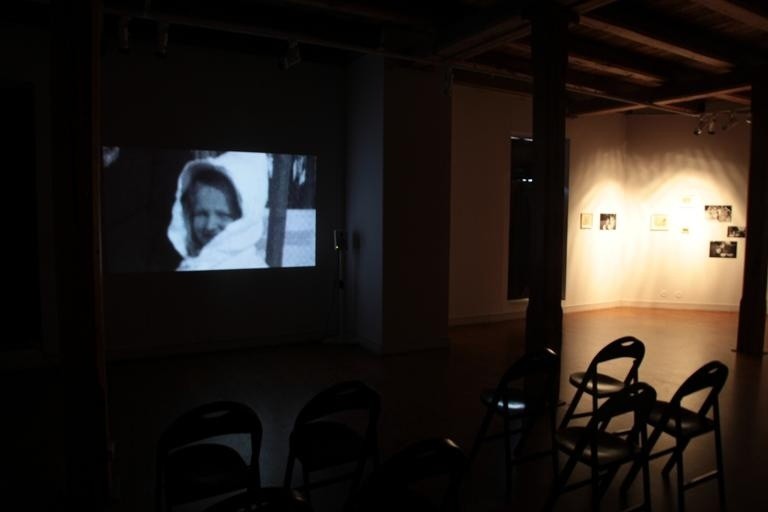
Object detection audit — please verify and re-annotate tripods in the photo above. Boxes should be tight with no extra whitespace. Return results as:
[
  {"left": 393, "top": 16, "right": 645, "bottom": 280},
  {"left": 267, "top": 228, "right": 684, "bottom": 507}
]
[{"left": 325, "top": 251, "right": 346, "bottom": 341}]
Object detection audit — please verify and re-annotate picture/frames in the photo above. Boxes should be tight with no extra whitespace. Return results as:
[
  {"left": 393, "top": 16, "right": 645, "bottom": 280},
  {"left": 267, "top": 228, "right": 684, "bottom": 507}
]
[
  {"left": 600, "top": 213, "right": 617, "bottom": 231},
  {"left": 650, "top": 202, "right": 747, "bottom": 258},
  {"left": 580, "top": 213, "right": 593, "bottom": 229}
]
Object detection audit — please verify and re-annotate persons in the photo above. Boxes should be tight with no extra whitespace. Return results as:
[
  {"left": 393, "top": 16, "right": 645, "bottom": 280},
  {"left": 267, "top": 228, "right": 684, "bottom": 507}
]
[{"left": 167, "top": 152, "right": 274, "bottom": 271}]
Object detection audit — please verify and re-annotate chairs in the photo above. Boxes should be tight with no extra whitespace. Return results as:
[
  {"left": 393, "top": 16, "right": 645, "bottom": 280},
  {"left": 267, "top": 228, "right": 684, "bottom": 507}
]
[
  {"left": 619, "top": 357, "right": 730, "bottom": 510},
  {"left": 157, "top": 399, "right": 267, "bottom": 510},
  {"left": 283, "top": 377, "right": 384, "bottom": 508},
  {"left": 542, "top": 382, "right": 655, "bottom": 510},
  {"left": 558, "top": 335, "right": 647, "bottom": 443},
  {"left": 470, "top": 344, "right": 567, "bottom": 497}
]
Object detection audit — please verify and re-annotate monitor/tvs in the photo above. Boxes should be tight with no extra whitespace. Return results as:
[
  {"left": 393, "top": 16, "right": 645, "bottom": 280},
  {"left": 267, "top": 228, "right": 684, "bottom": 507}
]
[{"left": 103, "top": 142, "right": 320, "bottom": 277}]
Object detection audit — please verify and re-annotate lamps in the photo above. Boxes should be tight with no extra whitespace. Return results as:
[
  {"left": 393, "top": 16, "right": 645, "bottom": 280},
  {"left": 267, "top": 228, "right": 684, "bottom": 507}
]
[{"left": 693, "top": 110, "right": 751, "bottom": 137}]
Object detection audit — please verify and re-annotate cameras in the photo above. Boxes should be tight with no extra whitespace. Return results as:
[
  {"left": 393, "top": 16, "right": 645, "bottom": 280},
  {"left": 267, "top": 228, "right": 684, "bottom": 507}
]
[{"left": 334, "top": 229, "right": 348, "bottom": 249}]
